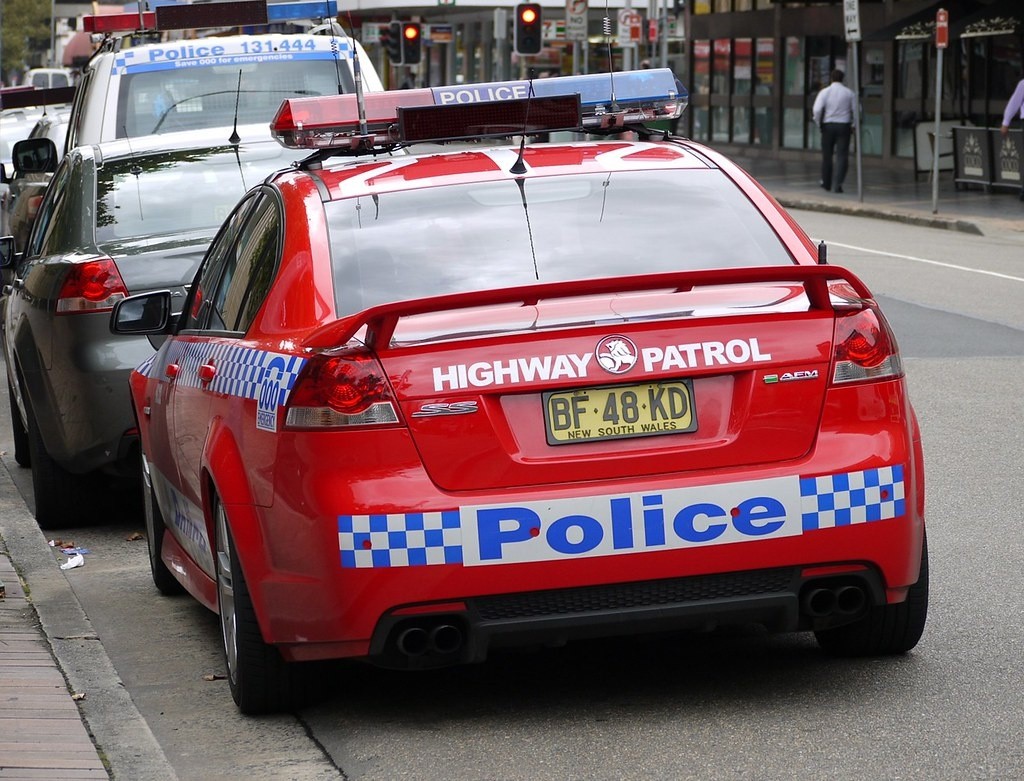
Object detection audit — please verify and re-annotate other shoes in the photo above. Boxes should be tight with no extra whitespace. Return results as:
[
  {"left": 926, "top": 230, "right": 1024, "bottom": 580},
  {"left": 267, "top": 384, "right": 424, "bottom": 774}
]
[
  {"left": 834, "top": 186, "right": 843, "bottom": 193},
  {"left": 818, "top": 179, "right": 831, "bottom": 190}
]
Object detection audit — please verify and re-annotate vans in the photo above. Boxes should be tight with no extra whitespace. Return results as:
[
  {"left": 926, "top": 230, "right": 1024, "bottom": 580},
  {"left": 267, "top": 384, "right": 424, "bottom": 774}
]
[
  {"left": 22, "top": 67, "right": 72, "bottom": 90},
  {"left": 12, "top": 0, "right": 384, "bottom": 174}
]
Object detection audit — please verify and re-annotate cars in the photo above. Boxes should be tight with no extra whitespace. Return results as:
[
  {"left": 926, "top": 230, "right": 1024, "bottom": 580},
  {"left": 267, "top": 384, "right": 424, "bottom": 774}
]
[
  {"left": 0, "top": 107, "right": 74, "bottom": 266},
  {"left": 109, "top": 65, "right": 930, "bottom": 721},
  {"left": 0, "top": 125, "right": 418, "bottom": 536}
]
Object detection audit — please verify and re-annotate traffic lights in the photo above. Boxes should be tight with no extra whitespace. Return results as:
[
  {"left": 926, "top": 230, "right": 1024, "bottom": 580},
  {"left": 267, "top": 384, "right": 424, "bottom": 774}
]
[
  {"left": 515, "top": 4, "right": 543, "bottom": 56},
  {"left": 401, "top": 22, "right": 421, "bottom": 65},
  {"left": 379, "top": 22, "right": 402, "bottom": 67}
]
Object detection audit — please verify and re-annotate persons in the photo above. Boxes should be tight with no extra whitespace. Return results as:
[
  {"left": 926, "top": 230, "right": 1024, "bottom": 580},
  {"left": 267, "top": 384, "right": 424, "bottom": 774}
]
[
  {"left": 812, "top": 68, "right": 863, "bottom": 194},
  {"left": 1001, "top": 79, "right": 1024, "bottom": 134},
  {"left": 745, "top": 78, "right": 771, "bottom": 143}
]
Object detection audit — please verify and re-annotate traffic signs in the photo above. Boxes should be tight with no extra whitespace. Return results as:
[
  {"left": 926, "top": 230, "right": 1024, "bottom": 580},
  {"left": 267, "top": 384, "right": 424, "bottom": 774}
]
[{"left": 843, "top": 0, "right": 861, "bottom": 41}]
